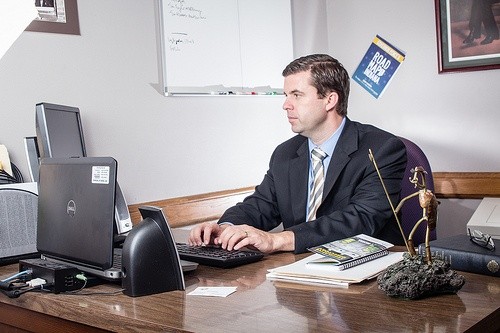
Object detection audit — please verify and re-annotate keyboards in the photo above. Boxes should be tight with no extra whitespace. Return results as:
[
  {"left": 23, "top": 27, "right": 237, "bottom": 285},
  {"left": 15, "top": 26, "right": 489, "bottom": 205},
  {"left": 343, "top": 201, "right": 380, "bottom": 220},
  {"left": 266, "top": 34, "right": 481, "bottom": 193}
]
[{"left": 176, "top": 242, "right": 265, "bottom": 268}]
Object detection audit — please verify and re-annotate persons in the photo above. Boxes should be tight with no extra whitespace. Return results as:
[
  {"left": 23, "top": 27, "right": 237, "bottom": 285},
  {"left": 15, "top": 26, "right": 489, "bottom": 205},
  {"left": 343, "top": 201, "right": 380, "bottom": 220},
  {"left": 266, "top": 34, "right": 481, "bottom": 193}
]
[
  {"left": 394, "top": 164, "right": 438, "bottom": 265},
  {"left": 187, "top": 52, "right": 408, "bottom": 255}
]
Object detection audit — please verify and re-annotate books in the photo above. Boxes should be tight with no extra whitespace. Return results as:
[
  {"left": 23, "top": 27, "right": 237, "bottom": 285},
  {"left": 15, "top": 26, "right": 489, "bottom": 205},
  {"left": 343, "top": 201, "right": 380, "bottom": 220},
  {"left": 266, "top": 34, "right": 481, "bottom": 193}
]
[
  {"left": 418, "top": 234, "right": 500, "bottom": 278},
  {"left": 265, "top": 233, "right": 409, "bottom": 288}
]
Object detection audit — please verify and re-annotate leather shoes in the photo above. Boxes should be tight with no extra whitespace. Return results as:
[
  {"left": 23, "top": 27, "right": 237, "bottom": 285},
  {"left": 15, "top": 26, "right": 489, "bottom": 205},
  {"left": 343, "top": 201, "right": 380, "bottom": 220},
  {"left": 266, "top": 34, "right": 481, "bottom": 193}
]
[
  {"left": 479, "top": 32, "right": 499, "bottom": 44},
  {"left": 463, "top": 30, "right": 481, "bottom": 43}
]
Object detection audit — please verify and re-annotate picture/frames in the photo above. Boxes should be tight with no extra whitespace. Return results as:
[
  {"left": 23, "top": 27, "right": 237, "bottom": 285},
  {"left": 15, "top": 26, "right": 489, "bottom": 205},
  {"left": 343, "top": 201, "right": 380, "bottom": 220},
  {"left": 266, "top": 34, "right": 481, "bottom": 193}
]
[{"left": 434, "top": 0, "right": 500, "bottom": 73}]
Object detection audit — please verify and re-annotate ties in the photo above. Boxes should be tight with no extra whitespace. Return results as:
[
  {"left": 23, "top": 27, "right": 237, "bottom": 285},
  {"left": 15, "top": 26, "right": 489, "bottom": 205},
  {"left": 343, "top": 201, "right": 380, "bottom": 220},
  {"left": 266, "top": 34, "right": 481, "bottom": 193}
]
[{"left": 306, "top": 147, "right": 328, "bottom": 223}]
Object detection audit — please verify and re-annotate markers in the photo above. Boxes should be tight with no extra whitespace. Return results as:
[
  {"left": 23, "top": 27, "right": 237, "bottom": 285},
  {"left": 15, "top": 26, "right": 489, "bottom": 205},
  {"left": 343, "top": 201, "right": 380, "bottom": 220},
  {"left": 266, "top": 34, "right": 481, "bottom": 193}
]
[
  {"left": 236, "top": 92, "right": 256, "bottom": 95},
  {"left": 211, "top": 91, "right": 233, "bottom": 95},
  {"left": 260, "top": 92, "right": 276, "bottom": 95}
]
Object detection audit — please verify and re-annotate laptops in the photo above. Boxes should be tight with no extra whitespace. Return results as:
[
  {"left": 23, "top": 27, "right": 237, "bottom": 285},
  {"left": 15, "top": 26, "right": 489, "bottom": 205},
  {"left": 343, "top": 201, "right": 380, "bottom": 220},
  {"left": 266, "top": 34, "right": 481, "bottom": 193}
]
[{"left": 36, "top": 157, "right": 198, "bottom": 281}]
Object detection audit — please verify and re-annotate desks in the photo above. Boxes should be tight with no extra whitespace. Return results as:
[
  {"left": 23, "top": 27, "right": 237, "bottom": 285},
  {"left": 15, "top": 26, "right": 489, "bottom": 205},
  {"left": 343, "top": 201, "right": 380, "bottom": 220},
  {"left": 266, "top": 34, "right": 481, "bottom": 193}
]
[{"left": 0, "top": 229, "right": 500, "bottom": 333}]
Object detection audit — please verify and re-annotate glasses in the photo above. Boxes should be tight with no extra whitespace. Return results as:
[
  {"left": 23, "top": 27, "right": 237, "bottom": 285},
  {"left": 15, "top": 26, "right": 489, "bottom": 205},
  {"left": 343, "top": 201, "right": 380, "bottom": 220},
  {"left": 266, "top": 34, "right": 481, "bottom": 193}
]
[{"left": 468, "top": 228, "right": 496, "bottom": 250}]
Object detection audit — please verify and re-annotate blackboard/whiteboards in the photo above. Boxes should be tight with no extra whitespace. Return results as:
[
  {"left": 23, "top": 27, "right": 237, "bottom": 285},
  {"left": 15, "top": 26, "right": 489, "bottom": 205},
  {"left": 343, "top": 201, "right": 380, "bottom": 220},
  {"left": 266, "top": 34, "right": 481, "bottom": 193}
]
[{"left": 159, "top": 0, "right": 293, "bottom": 96}]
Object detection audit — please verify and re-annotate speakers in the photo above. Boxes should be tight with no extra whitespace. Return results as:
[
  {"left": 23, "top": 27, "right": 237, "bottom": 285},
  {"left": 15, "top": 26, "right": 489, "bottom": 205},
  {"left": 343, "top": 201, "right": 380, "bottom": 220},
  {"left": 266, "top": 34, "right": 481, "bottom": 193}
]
[
  {"left": 114, "top": 181, "right": 133, "bottom": 235},
  {"left": 121, "top": 204, "right": 185, "bottom": 298}
]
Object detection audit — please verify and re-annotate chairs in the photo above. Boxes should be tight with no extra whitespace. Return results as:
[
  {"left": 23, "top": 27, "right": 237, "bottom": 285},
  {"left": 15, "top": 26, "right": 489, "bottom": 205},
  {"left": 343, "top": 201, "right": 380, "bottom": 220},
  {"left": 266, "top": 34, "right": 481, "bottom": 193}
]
[{"left": 399, "top": 136, "right": 436, "bottom": 246}]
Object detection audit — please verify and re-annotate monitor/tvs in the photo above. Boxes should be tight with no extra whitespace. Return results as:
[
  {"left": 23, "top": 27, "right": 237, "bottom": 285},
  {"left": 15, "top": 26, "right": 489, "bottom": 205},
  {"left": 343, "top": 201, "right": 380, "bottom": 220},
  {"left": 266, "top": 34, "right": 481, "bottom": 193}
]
[{"left": 24, "top": 102, "right": 87, "bottom": 184}]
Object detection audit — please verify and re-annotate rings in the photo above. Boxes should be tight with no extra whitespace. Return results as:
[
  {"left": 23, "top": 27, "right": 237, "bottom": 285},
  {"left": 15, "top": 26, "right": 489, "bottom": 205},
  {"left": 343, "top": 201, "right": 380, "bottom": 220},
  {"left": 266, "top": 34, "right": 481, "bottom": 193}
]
[{"left": 245, "top": 232, "right": 248, "bottom": 236}]
